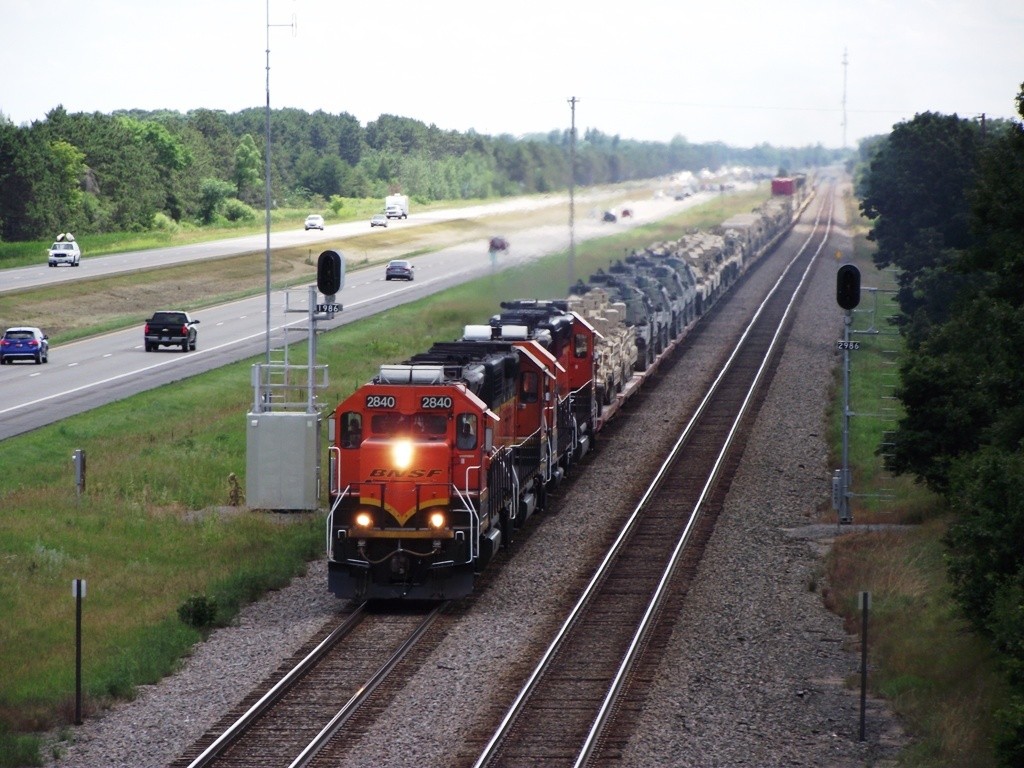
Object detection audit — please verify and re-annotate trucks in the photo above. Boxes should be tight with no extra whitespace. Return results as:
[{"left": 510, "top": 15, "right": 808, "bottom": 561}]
[{"left": 385, "top": 193, "right": 408, "bottom": 220}]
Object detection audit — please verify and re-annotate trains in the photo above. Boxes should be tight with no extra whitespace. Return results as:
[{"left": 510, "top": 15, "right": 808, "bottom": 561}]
[{"left": 326, "top": 169, "right": 804, "bottom": 610}]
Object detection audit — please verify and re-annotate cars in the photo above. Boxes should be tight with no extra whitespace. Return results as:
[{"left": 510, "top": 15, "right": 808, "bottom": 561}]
[
  {"left": 489, "top": 237, "right": 509, "bottom": 250},
  {"left": 603, "top": 201, "right": 633, "bottom": 224},
  {"left": 385, "top": 260, "right": 414, "bottom": 280},
  {"left": 305, "top": 215, "right": 324, "bottom": 230},
  {"left": 370, "top": 214, "right": 388, "bottom": 227}
]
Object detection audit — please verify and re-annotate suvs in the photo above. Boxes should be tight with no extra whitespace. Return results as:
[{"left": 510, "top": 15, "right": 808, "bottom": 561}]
[
  {"left": 0, "top": 327, "right": 47, "bottom": 364},
  {"left": 144, "top": 311, "right": 196, "bottom": 352},
  {"left": 48, "top": 232, "right": 80, "bottom": 268}
]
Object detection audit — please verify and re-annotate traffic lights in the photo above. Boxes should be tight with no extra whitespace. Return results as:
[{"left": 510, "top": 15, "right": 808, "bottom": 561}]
[
  {"left": 836, "top": 263, "right": 861, "bottom": 310},
  {"left": 317, "top": 249, "right": 342, "bottom": 295}
]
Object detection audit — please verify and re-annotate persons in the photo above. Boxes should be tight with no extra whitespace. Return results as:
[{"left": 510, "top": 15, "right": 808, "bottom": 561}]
[{"left": 342, "top": 418, "right": 362, "bottom": 448}]
